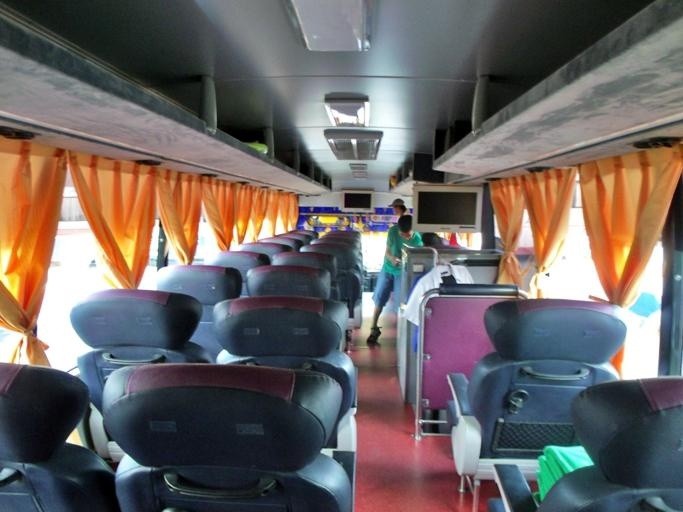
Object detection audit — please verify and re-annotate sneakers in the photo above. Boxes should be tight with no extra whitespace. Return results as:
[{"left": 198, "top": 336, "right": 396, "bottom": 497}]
[{"left": 367, "top": 328, "right": 381, "bottom": 343}]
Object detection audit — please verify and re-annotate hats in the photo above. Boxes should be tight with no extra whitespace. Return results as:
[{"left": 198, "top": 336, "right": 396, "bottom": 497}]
[{"left": 388, "top": 199, "right": 405, "bottom": 207}]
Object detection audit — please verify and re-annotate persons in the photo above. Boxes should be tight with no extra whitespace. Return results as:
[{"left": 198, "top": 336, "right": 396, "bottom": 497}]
[
  {"left": 388, "top": 197, "right": 413, "bottom": 217},
  {"left": 422, "top": 231, "right": 443, "bottom": 248},
  {"left": 367, "top": 215, "right": 424, "bottom": 344}
]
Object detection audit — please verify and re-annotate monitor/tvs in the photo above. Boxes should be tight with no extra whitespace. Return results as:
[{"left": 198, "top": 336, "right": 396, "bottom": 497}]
[
  {"left": 341, "top": 190, "right": 375, "bottom": 213},
  {"left": 411, "top": 183, "right": 484, "bottom": 233}
]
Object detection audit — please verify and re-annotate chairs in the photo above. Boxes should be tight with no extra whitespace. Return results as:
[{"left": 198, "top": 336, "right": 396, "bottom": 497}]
[
  {"left": 487, "top": 377, "right": 683, "bottom": 511},
  {"left": 447, "top": 300, "right": 627, "bottom": 493},
  {"left": 1, "top": 228, "right": 363, "bottom": 512}
]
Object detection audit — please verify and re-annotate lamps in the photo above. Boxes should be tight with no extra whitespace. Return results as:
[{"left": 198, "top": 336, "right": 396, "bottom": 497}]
[
  {"left": 282, "top": 0, "right": 374, "bottom": 53},
  {"left": 324, "top": 99, "right": 370, "bottom": 128}
]
